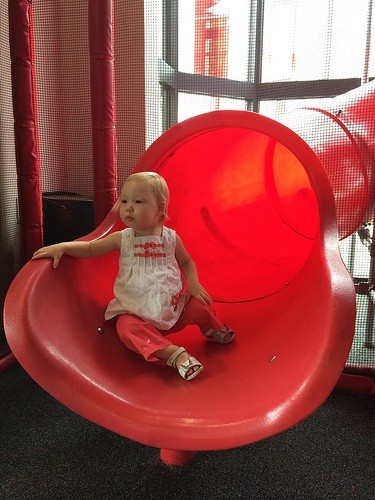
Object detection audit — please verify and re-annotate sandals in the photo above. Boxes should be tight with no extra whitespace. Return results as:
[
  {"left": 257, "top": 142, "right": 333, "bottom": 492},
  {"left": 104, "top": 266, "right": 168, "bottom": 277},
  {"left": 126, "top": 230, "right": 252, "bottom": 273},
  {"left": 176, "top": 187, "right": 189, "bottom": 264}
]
[
  {"left": 166, "top": 347, "right": 203, "bottom": 380},
  {"left": 202, "top": 324, "right": 235, "bottom": 344}
]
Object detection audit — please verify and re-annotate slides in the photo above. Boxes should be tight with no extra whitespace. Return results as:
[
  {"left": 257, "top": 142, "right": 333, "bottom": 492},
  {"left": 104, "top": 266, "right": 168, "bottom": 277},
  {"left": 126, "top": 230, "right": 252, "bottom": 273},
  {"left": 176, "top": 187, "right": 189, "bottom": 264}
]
[{"left": 3, "top": 78, "right": 375, "bottom": 465}]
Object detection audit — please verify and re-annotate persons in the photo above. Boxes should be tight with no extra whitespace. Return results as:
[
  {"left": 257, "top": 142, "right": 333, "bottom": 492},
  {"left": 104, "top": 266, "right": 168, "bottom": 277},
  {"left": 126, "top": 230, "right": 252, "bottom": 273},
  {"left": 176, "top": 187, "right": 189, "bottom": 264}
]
[{"left": 30, "top": 172, "right": 235, "bottom": 383}]
